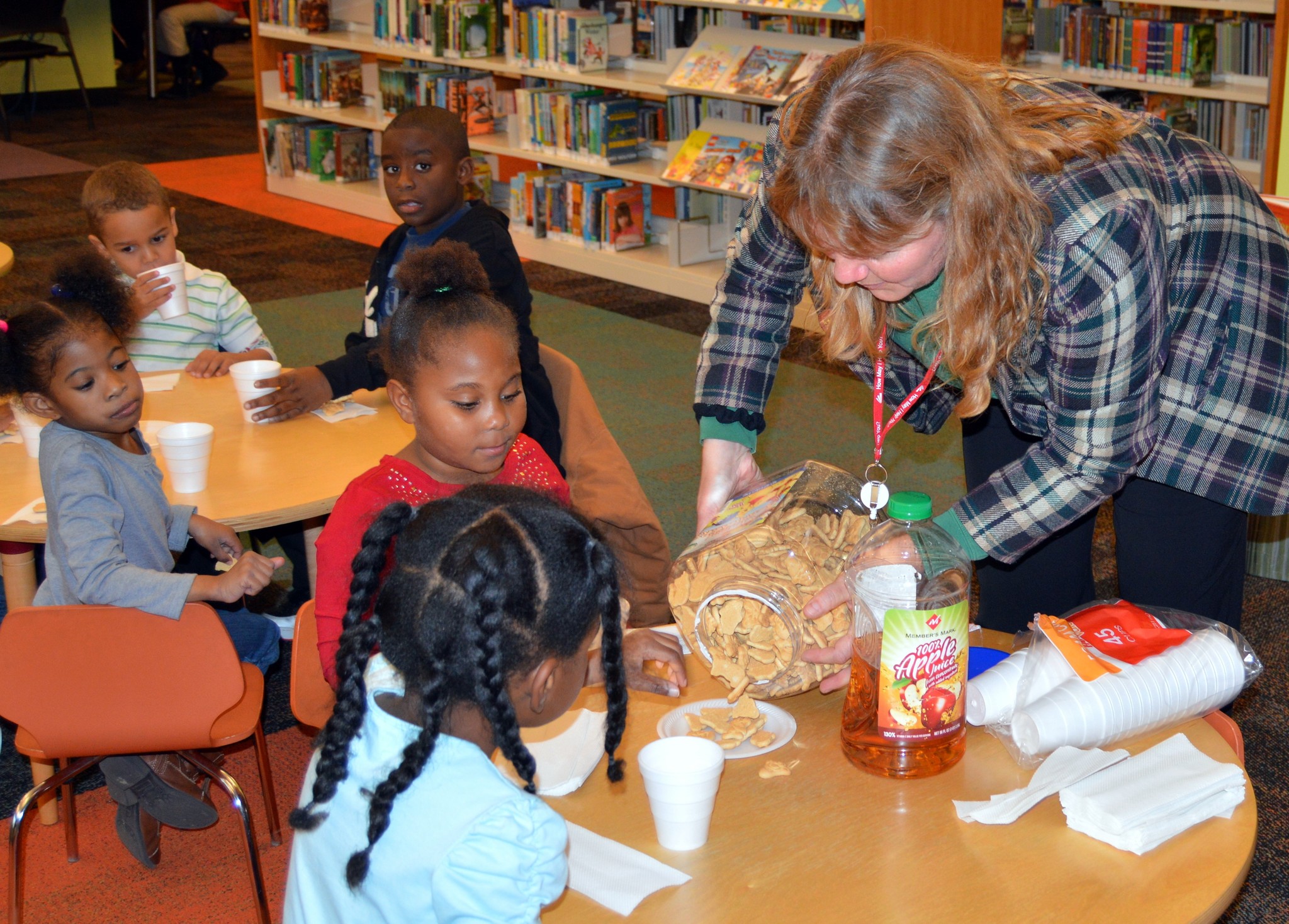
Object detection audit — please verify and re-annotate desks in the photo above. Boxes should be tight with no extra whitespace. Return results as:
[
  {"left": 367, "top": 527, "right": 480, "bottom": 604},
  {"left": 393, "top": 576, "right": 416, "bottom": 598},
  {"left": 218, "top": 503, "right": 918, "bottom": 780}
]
[
  {"left": 492, "top": 627, "right": 1258, "bottom": 924},
  {"left": 0, "top": 370, "right": 416, "bottom": 606}
]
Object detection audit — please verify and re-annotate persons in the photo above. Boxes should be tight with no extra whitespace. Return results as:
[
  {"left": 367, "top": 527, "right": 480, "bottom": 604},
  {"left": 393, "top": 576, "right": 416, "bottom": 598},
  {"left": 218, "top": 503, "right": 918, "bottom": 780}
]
[
  {"left": 0, "top": 97, "right": 633, "bottom": 924},
  {"left": 152, "top": 0, "right": 246, "bottom": 101},
  {"left": 693, "top": 41, "right": 1289, "bottom": 726}
]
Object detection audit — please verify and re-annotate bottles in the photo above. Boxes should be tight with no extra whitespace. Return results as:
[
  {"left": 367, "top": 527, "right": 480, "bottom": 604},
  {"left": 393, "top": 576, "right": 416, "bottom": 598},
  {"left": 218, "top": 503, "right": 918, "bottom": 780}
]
[
  {"left": 667, "top": 459, "right": 889, "bottom": 699},
  {"left": 838, "top": 490, "right": 973, "bottom": 780}
]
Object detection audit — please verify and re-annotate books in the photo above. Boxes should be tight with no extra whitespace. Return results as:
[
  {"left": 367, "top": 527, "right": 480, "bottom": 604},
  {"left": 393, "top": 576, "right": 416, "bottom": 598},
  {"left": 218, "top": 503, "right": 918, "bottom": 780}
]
[{"left": 254, "top": 0, "right": 1274, "bottom": 274}]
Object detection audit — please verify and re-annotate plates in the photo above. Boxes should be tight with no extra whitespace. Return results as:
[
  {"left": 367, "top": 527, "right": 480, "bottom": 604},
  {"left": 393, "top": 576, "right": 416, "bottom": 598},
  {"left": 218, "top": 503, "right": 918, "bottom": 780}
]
[
  {"left": 659, "top": 699, "right": 798, "bottom": 758},
  {"left": 138, "top": 420, "right": 172, "bottom": 448}
]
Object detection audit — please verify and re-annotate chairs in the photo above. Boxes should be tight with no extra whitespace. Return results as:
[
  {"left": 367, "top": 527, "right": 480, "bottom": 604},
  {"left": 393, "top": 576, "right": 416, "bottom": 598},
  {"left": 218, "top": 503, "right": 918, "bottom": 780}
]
[
  {"left": 0, "top": 0, "right": 95, "bottom": 142},
  {"left": 0, "top": 601, "right": 282, "bottom": 924}
]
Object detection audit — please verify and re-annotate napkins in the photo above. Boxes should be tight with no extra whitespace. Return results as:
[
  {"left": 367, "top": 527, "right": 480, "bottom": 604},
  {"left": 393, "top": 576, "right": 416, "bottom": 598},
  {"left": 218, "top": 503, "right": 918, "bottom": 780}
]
[
  {"left": 493, "top": 709, "right": 693, "bottom": 917},
  {"left": 952, "top": 733, "right": 1247, "bottom": 856}
]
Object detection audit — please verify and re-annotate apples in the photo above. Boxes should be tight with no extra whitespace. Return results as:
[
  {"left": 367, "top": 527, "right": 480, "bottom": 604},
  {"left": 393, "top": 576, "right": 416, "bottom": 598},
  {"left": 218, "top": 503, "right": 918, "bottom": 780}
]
[{"left": 889, "top": 674, "right": 963, "bottom": 730}]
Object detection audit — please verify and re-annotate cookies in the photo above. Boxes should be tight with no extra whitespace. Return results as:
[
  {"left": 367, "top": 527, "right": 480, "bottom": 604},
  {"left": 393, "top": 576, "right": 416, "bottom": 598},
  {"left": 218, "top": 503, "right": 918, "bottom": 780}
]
[
  {"left": 685, "top": 694, "right": 775, "bottom": 750},
  {"left": 32, "top": 502, "right": 47, "bottom": 513},
  {"left": 0, "top": 423, "right": 19, "bottom": 436},
  {"left": 758, "top": 759, "right": 791, "bottom": 778},
  {"left": 668, "top": 492, "right": 873, "bottom": 704},
  {"left": 215, "top": 553, "right": 238, "bottom": 572},
  {"left": 322, "top": 393, "right": 355, "bottom": 416}
]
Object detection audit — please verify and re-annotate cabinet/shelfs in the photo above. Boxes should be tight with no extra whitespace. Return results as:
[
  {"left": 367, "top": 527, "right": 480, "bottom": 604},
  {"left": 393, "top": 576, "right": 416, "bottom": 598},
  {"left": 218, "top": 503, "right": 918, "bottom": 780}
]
[{"left": 248, "top": 0, "right": 1289, "bottom": 335}]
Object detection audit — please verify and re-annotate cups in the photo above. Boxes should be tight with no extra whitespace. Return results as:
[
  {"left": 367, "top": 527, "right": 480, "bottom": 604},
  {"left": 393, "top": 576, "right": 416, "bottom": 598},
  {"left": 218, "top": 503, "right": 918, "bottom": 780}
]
[
  {"left": 8, "top": 396, "right": 52, "bottom": 458},
  {"left": 230, "top": 359, "right": 283, "bottom": 423},
  {"left": 136, "top": 263, "right": 190, "bottom": 320},
  {"left": 637, "top": 736, "right": 727, "bottom": 852},
  {"left": 965, "top": 616, "right": 1246, "bottom": 756},
  {"left": 156, "top": 421, "right": 213, "bottom": 495}
]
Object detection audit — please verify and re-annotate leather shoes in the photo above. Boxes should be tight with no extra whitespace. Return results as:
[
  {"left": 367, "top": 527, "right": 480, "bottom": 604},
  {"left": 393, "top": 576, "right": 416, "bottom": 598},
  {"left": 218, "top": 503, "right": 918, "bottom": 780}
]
[
  {"left": 105, "top": 775, "right": 161, "bottom": 868},
  {"left": 100, "top": 754, "right": 218, "bottom": 829}
]
